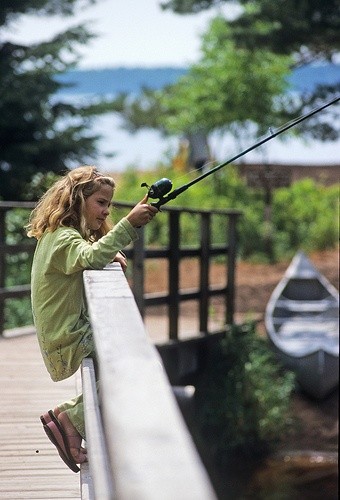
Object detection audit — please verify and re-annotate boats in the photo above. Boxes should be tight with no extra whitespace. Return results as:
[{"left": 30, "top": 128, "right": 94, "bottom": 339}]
[{"left": 264, "top": 248, "right": 340, "bottom": 401}]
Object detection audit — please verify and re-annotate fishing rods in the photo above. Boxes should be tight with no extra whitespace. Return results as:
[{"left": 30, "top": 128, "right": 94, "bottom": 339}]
[{"left": 141, "top": 93, "right": 340, "bottom": 213}]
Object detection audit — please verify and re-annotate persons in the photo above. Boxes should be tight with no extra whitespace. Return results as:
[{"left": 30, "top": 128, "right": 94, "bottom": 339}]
[{"left": 23, "top": 164, "right": 159, "bottom": 474}]
